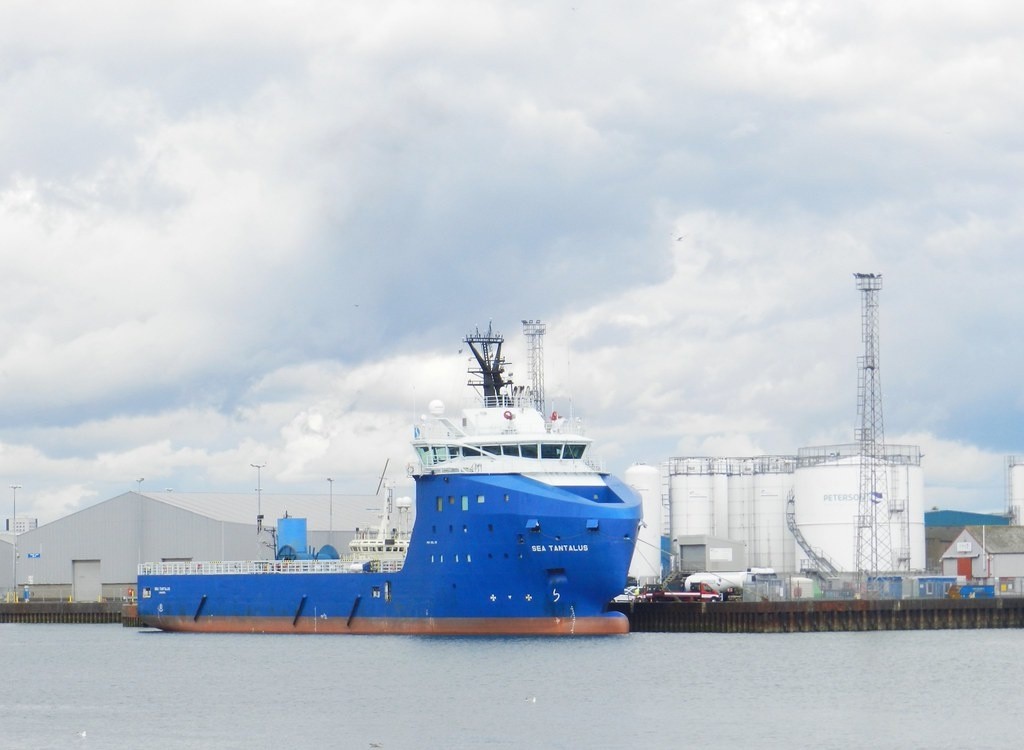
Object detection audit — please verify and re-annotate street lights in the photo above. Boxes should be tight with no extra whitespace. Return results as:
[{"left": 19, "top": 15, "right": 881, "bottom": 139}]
[
  {"left": 10, "top": 484, "right": 22, "bottom": 593},
  {"left": 326, "top": 476, "right": 335, "bottom": 546},
  {"left": 250, "top": 463, "right": 265, "bottom": 535}
]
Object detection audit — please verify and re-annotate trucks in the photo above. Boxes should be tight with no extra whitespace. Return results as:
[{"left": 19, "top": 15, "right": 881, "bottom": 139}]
[{"left": 646, "top": 581, "right": 721, "bottom": 603}]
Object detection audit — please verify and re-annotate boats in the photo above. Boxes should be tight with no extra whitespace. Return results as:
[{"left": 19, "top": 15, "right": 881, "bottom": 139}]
[{"left": 136, "top": 319, "right": 647, "bottom": 636}]
